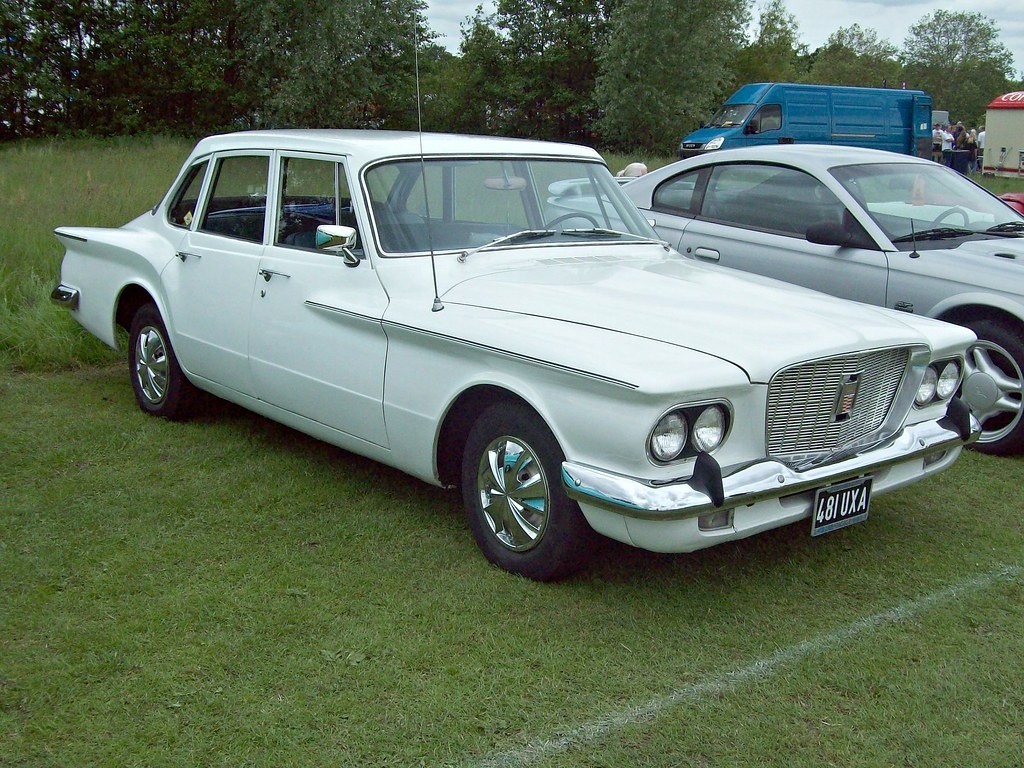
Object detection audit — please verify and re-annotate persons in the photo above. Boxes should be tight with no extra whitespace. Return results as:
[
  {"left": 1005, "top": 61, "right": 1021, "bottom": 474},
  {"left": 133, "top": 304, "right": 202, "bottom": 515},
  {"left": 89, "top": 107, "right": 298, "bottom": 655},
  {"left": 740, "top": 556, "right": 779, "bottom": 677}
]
[
  {"left": 932, "top": 121, "right": 985, "bottom": 175},
  {"left": 942, "top": 125, "right": 954, "bottom": 167}
]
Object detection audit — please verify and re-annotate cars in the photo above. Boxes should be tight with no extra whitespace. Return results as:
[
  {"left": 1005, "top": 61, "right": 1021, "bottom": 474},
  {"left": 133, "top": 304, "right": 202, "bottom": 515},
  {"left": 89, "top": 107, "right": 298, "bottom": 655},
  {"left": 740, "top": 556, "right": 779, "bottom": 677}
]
[
  {"left": 540, "top": 142, "right": 1023, "bottom": 458},
  {"left": 52, "top": 131, "right": 983, "bottom": 582}
]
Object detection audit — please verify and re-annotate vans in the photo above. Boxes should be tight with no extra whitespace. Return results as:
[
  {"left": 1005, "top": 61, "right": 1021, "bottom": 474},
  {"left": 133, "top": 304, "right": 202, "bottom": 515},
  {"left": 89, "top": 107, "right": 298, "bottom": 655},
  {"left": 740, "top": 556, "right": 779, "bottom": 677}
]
[{"left": 679, "top": 83, "right": 932, "bottom": 162}]
